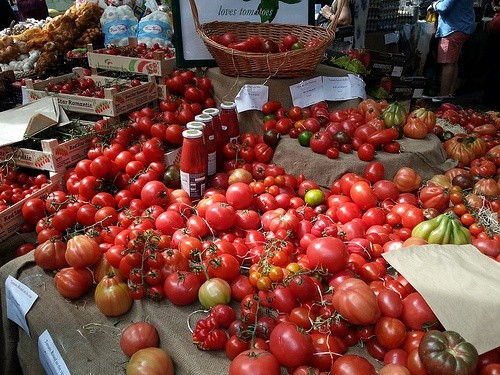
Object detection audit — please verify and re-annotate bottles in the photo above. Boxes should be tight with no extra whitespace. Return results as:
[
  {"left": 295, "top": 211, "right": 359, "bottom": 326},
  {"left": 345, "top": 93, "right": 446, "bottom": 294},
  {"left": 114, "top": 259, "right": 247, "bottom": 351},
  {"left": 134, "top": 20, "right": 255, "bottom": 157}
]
[{"left": 180, "top": 102, "right": 240, "bottom": 204}]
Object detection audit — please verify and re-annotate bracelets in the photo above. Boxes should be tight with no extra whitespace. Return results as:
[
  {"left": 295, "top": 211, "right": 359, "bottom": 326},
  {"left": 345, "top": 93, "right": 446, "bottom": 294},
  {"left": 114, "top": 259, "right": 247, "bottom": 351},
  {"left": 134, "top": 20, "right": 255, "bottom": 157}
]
[{"left": 329, "top": 13, "right": 334, "bottom": 19}]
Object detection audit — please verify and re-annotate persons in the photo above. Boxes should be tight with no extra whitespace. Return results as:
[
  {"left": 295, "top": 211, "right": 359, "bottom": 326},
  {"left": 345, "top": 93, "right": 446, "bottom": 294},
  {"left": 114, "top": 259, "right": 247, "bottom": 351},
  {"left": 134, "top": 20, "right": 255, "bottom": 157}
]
[
  {"left": 316, "top": 0, "right": 353, "bottom": 51},
  {"left": 10, "top": 0, "right": 51, "bottom": 24},
  {"left": 427, "top": 0, "right": 476, "bottom": 95}
]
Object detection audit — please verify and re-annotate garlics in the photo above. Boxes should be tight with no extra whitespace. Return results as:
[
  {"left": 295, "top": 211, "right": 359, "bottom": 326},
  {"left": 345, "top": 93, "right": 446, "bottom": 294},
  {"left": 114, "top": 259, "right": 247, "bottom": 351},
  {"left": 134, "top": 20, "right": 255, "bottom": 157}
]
[
  {"left": 0, "top": 3, "right": 99, "bottom": 72},
  {"left": 100, "top": 5, "right": 172, "bottom": 48}
]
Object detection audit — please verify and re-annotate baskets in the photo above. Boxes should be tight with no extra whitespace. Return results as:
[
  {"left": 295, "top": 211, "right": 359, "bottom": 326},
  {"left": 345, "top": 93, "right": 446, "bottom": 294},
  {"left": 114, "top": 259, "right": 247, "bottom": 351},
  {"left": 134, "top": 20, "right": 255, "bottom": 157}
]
[{"left": 189, "top": 0, "right": 344, "bottom": 79}]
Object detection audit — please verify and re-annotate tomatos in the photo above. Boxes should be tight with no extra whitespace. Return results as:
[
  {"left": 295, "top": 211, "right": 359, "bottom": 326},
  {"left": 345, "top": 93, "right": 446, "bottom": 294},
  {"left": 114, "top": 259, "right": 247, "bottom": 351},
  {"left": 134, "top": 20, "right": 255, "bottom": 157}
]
[
  {"left": 0, "top": 43, "right": 500, "bottom": 374},
  {"left": 210, "top": 33, "right": 318, "bottom": 53}
]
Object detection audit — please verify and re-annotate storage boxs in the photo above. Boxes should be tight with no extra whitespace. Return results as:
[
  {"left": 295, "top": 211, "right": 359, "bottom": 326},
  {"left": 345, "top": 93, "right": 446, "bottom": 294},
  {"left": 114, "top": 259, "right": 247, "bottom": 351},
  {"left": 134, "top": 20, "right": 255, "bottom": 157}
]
[
  {"left": 322, "top": 47, "right": 455, "bottom": 112},
  {"left": 0, "top": 44, "right": 177, "bottom": 242}
]
